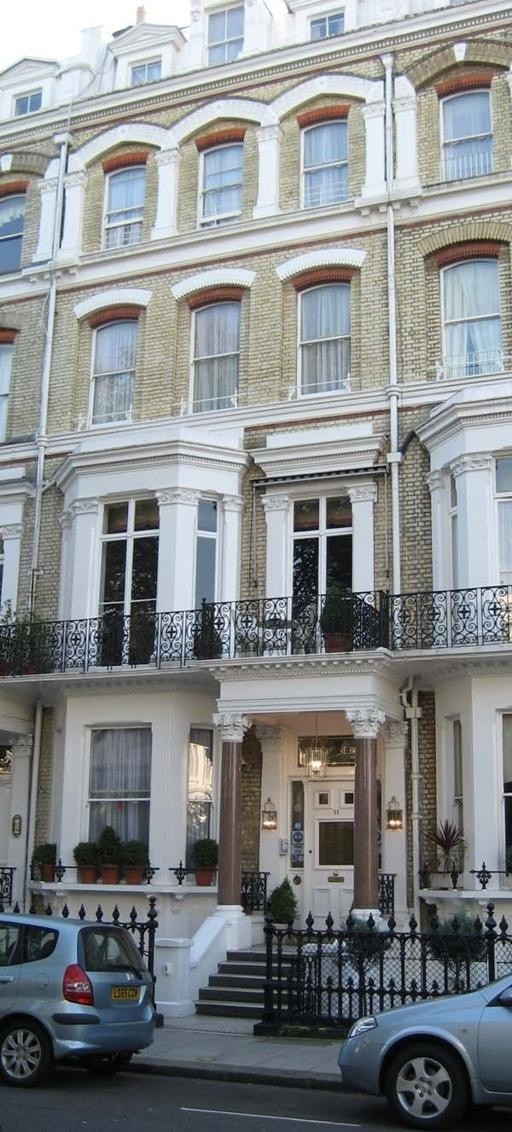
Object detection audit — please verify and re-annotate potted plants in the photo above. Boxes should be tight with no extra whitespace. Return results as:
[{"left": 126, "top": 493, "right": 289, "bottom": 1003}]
[
  {"left": 71, "top": 841, "right": 98, "bottom": 883},
  {"left": 190, "top": 836, "right": 218, "bottom": 886},
  {"left": 318, "top": 585, "right": 355, "bottom": 652},
  {"left": 35, "top": 845, "right": 55, "bottom": 881},
  {"left": 0, "top": 597, "right": 22, "bottom": 674},
  {"left": 265, "top": 876, "right": 299, "bottom": 943},
  {"left": 421, "top": 816, "right": 470, "bottom": 917},
  {"left": 20, "top": 607, "right": 57, "bottom": 674},
  {"left": 119, "top": 839, "right": 148, "bottom": 885},
  {"left": 96, "top": 825, "right": 123, "bottom": 883}
]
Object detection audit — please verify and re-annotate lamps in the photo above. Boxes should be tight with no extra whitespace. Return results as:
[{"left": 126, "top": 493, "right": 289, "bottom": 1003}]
[
  {"left": 302, "top": 712, "right": 327, "bottom": 781},
  {"left": 261, "top": 796, "right": 279, "bottom": 831},
  {"left": 385, "top": 795, "right": 404, "bottom": 832}
]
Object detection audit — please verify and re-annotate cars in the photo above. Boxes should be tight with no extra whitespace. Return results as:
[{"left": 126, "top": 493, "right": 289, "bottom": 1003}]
[
  {"left": 0, "top": 907, "right": 163, "bottom": 1093},
  {"left": 333, "top": 964, "right": 512, "bottom": 1125}
]
